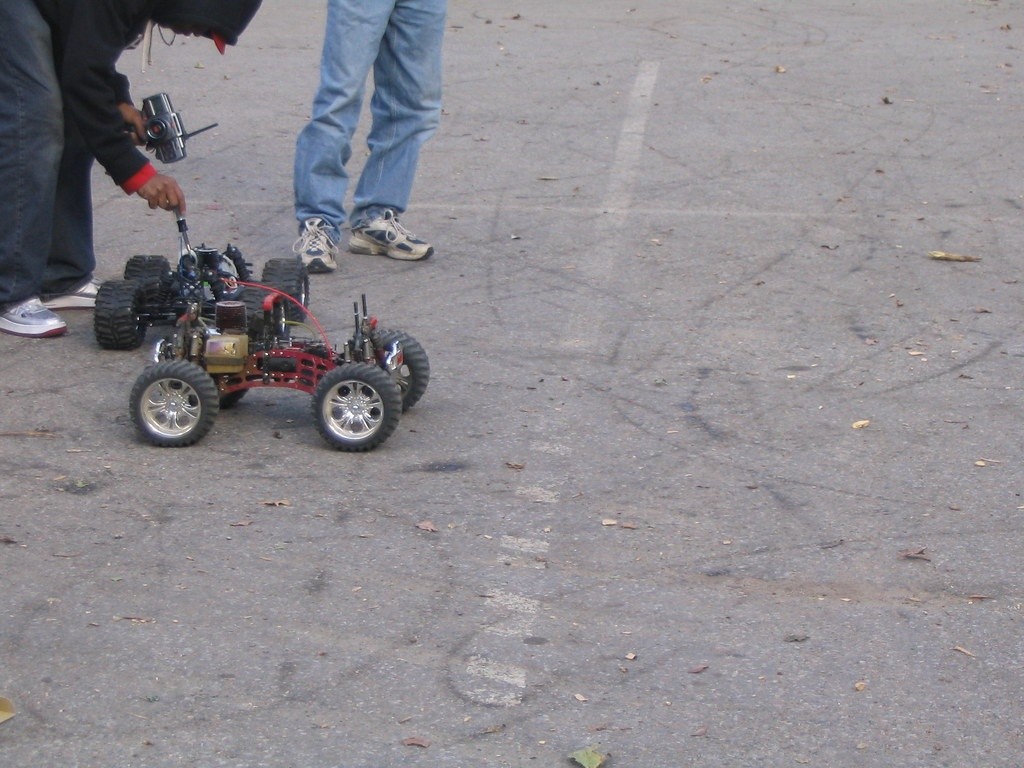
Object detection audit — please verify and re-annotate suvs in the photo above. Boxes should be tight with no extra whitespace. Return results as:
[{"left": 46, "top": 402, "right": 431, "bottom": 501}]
[
  {"left": 92, "top": 241, "right": 311, "bottom": 352},
  {"left": 127, "top": 293, "right": 430, "bottom": 454}
]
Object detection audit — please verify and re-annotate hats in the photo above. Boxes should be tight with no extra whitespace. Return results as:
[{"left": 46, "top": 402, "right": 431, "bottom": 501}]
[{"left": 211, "top": 28, "right": 226, "bottom": 56}]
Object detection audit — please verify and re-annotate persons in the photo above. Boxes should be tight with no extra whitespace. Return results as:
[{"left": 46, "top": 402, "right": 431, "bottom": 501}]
[
  {"left": 0, "top": 0, "right": 266, "bottom": 338},
  {"left": 292, "top": 0, "right": 444, "bottom": 272}
]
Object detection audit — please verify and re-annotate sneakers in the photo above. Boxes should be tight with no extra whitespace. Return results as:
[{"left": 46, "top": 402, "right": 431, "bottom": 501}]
[
  {"left": 0, "top": 296, "right": 68, "bottom": 338},
  {"left": 347, "top": 210, "right": 435, "bottom": 262},
  {"left": 40, "top": 277, "right": 104, "bottom": 311},
  {"left": 291, "top": 217, "right": 339, "bottom": 274}
]
